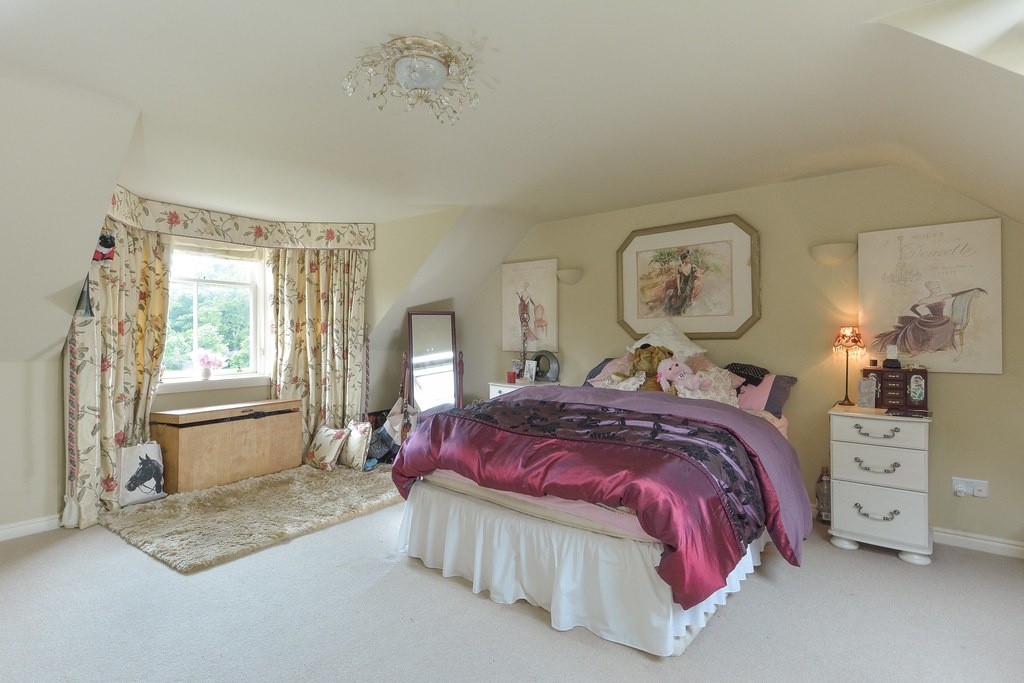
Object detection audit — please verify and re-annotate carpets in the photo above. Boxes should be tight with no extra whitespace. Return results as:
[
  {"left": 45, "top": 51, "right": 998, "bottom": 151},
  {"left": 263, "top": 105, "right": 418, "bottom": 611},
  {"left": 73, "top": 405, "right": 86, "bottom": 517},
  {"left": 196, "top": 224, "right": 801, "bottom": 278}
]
[{"left": 96, "top": 459, "right": 418, "bottom": 576}]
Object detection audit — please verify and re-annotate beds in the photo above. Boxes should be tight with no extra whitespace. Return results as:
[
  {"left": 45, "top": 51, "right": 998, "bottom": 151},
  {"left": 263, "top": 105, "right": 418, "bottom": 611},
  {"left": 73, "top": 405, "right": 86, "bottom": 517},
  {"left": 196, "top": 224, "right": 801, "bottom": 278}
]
[{"left": 390, "top": 385, "right": 812, "bottom": 657}]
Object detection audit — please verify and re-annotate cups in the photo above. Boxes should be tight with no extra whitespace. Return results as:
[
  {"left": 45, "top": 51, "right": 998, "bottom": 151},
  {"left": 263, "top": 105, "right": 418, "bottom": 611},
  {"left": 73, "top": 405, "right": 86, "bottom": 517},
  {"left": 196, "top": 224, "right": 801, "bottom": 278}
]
[{"left": 507, "top": 372, "right": 516, "bottom": 382}]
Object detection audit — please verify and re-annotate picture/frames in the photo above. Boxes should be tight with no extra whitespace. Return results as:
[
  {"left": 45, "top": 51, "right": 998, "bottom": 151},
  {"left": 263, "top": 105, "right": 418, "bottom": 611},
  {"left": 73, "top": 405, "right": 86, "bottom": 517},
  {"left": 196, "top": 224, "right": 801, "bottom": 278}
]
[{"left": 614, "top": 212, "right": 761, "bottom": 341}]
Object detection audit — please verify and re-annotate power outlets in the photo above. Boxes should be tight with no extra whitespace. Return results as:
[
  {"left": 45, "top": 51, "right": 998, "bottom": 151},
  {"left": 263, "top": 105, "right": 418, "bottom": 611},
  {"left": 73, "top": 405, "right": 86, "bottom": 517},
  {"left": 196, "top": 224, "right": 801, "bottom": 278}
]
[{"left": 951, "top": 477, "right": 989, "bottom": 499}]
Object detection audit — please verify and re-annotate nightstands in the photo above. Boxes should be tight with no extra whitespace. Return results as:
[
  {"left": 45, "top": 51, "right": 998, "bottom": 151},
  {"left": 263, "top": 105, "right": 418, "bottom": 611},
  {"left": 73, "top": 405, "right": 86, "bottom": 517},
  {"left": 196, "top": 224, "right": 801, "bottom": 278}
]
[
  {"left": 488, "top": 382, "right": 559, "bottom": 400},
  {"left": 827, "top": 404, "right": 934, "bottom": 566}
]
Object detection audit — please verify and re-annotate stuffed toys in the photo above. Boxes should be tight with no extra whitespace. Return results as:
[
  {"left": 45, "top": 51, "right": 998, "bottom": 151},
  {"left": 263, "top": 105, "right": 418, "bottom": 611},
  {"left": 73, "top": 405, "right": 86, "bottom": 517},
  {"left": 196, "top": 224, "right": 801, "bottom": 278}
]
[
  {"left": 656, "top": 356, "right": 712, "bottom": 397},
  {"left": 611, "top": 344, "right": 678, "bottom": 395}
]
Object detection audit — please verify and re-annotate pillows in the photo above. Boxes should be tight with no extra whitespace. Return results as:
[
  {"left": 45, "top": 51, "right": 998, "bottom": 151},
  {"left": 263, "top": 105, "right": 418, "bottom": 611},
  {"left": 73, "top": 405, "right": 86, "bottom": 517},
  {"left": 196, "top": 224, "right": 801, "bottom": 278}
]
[
  {"left": 583, "top": 322, "right": 797, "bottom": 419},
  {"left": 305, "top": 419, "right": 399, "bottom": 470},
  {"left": 118, "top": 442, "right": 170, "bottom": 506}
]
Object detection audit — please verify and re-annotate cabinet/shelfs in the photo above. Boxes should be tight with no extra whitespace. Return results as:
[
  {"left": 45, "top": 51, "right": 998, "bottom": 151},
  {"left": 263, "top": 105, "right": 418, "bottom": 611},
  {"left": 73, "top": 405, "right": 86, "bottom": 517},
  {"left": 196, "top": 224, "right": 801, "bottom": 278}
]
[{"left": 863, "top": 368, "right": 928, "bottom": 417}]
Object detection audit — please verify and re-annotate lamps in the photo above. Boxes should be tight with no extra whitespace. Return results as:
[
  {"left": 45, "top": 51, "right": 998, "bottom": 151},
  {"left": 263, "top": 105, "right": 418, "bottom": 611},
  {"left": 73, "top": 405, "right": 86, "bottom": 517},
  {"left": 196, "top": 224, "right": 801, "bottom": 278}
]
[
  {"left": 833, "top": 327, "right": 866, "bottom": 405},
  {"left": 557, "top": 268, "right": 584, "bottom": 284},
  {"left": 341, "top": 34, "right": 478, "bottom": 129},
  {"left": 808, "top": 242, "right": 857, "bottom": 264}
]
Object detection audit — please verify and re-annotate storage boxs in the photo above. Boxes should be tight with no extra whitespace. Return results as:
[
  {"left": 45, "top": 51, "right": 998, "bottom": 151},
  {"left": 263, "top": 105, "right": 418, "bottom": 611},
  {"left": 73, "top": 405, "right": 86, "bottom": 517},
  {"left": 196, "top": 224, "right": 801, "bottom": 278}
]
[{"left": 148, "top": 398, "right": 303, "bottom": 494}]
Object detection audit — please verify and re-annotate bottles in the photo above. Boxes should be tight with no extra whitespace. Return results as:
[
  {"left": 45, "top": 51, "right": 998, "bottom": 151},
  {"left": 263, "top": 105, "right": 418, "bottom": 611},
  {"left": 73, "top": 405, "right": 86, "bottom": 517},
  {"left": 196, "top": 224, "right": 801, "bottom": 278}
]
[
  {"left": 815, "top": 466, "right": 831, "bottom": 524},
  {"left": 402, "top": 423, "right": 412, "bottom": 443}
]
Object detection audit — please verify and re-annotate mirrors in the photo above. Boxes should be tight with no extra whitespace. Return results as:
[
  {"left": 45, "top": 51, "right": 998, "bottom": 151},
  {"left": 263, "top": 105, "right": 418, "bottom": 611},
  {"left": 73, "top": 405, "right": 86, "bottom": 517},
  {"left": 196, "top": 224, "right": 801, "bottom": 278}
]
[{"left": 401, "top": 311, "right": 463, "bottom": 411}]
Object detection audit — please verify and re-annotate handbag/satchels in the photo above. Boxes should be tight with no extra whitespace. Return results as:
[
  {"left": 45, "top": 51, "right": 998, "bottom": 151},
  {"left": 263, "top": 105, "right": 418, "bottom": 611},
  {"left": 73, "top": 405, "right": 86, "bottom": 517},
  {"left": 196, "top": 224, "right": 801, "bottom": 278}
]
[
  {"left": 117, "top": 440, "right": 167, "bottom": 508},
  {"left": 379, "top": 397, "right": 417, "bottom": 453}
]
